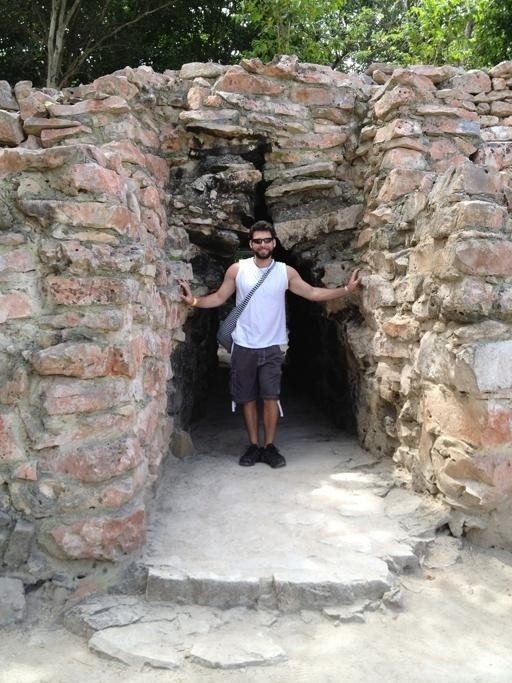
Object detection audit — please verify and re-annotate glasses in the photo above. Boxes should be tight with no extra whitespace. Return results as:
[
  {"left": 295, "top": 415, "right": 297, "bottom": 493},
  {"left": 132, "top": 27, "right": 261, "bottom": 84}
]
[{"left": 250, "top": 238, "right": 274, "bottom": 244}]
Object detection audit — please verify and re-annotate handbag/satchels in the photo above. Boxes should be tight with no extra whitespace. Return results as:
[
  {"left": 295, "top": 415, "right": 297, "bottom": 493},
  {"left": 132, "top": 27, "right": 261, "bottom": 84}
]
[{"left": 214, "top": 306, "right": 242, "bottom": 352}]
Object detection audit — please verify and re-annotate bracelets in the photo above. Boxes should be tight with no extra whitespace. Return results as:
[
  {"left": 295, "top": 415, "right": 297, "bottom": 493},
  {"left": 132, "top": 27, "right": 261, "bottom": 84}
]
[
  {"left": 343, "top": 285, "right": 351, "bottom": 296},
  {"left": 190, "top": 297, "right": 198, "bottom": 308}
]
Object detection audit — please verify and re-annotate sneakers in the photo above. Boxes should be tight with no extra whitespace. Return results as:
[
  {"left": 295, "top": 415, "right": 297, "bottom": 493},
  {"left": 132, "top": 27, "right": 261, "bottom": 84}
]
[
  {"left": 239, "top": 444, "right": 262, "bottom": 466},
  {"left": 263, "top": 442, "right": 286, "bottom": 468}
]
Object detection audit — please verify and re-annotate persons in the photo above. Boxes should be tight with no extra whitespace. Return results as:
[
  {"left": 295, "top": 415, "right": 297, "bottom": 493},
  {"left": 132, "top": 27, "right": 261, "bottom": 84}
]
[{"left": 177, "top": 220, "right": 363, "bottom": 469}]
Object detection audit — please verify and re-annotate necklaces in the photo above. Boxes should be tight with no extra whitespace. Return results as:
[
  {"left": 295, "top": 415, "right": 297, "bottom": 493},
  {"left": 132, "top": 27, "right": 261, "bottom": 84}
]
[{"left": 253, "top": 257, "right": 272, "bottom": 268}]
